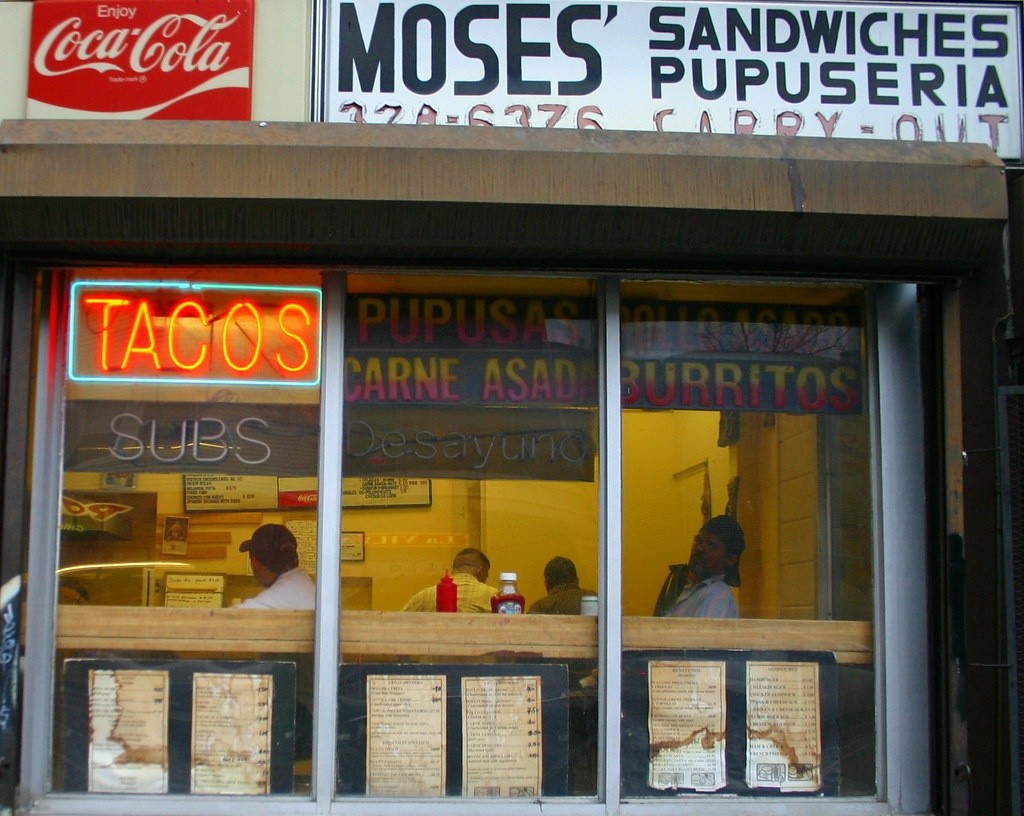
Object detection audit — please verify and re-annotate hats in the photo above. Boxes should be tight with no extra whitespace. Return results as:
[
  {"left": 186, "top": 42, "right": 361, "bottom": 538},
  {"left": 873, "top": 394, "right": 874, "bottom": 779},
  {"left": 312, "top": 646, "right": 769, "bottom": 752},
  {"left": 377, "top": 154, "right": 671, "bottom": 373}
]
[
  {"left": 699, "top": 515, "right": 745, "bottom": 586},
  {"left": 240, "top": 523, "right": 297, "bottom": 566}
]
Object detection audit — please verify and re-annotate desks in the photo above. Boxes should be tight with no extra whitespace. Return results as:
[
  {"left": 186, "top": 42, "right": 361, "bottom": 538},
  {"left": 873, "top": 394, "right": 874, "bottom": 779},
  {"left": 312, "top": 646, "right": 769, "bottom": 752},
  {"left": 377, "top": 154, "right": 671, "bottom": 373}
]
[{"left": 15, "top": 601, "right": 877, "bottom": 800}]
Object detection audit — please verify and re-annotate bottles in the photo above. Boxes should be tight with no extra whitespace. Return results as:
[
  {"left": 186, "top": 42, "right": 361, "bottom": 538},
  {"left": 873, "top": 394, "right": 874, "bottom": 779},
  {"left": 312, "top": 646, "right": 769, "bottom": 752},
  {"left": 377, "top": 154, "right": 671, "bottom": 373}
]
[
  {"left": 436, "top": 568, "right": 459, "bottom": 613},
  {"left": 490, "top": 573, "right": 525, "bottom": 615}
]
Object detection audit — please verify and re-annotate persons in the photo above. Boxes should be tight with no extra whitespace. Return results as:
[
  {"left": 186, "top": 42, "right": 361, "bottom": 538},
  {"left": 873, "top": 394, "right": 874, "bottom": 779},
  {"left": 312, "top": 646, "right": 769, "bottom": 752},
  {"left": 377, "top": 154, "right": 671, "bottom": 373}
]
[
  {"left": 228, "top": 523, "right": 317, "bottom": 610},
  {"left": 660, "top": 516, "right": 745, "bottom": 620},
  {"left": 400, "top": 547, "right": 502, "bottom": 614},
  {"left": 525, "top": 556, "right": 598, "bottom": 616}
]
